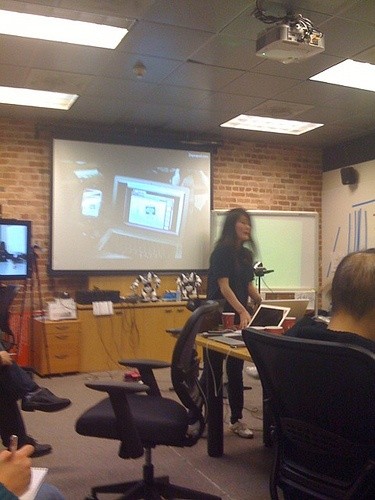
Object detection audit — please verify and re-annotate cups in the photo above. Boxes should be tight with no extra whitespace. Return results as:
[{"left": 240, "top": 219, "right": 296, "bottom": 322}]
[
  {"left": 282, "top": 318, "right": 296, "bottom": 335},
  {"left": 221, "top": 312, "right": 235, "bottom": 330}
]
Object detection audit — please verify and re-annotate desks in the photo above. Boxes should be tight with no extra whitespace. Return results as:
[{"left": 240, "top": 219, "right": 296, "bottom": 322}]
[{"left": 172, "top": 330, "right": 255, "bottom": 458}]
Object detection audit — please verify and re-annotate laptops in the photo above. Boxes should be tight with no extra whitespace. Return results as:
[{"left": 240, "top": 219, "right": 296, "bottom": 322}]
[
  {"left": 208, "top": 304, "right": 291, "bottom": 346},
  {"left": 260, "top": 299, "right": 309, "bottom": 324}
]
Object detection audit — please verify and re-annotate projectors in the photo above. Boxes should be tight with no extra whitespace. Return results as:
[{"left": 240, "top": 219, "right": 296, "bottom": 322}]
[{"left": 255, "top": 20, "right": 325, "bottom": 65}]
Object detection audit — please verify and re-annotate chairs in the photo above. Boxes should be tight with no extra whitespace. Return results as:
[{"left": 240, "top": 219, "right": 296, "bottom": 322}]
[{"left": 75, "top": 303, "right": 375, "bottom": 500}]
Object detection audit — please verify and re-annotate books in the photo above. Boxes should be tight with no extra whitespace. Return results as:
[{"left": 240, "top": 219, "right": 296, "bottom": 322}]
[{"left": 17, "top": 467, "right": 48, "bottom": 500}]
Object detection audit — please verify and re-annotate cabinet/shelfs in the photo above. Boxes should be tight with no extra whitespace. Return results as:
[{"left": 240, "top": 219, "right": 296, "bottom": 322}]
[{"left": 35, "top": 303, "right": 201, "bottom": 376}]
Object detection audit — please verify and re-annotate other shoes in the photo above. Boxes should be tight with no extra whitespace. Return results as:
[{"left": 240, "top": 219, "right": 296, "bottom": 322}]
[
  {"left": 229, "top": 420, "right": 254, "bottom": 439},
  {"left": 201, "top": 423, "right": 209, "bottom": 439}
]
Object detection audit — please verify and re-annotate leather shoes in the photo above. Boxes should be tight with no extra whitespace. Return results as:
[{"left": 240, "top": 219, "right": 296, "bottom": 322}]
[
  {"left": 21, "top": 387, "right": 71, "bottom": 413},
  {"left": 3, "top": 434, "right": 52, "bottom": 458}
]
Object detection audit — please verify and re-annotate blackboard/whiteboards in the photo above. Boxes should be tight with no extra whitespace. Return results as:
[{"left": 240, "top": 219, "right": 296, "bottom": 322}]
[{"left": 212, "top": 209, "right": 320, "bottom": 293}]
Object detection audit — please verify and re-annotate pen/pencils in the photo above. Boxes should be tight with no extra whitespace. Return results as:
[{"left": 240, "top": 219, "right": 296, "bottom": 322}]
[
  {"left": 8, "top": 353, "right": 16, "bottom": 355},
  {"left": 10, "top": 435, "right": 18, "bottom": 451}
]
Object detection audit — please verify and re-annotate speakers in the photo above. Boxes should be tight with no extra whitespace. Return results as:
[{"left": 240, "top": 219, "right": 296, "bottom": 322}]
[{"left": 341, "top": 167, "right": 357, "bottom": 186}]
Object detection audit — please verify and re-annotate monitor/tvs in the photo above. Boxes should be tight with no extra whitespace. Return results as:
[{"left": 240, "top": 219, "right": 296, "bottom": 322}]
[
  {"left": 80, "top": 188, "right": 103, "bottom": 219},
  {"left": 0, "top": 219, "right": 31, "bottom": 280},
  {"left": 112, "top": 174, "right": 191, "bottom": 248}
]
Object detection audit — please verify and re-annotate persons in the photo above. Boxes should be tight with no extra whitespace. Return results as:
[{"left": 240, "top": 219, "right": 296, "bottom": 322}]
[
  {"left": 287, "top": 251, "right": 375, "bottom": 354},
  {"left": 0, "top": 445, "right": 67, "bottom": 500},
  {"left": 0, "top": 343, "right": 71, "bottom": 457},
  {"left": 203, "top": 208, "right": 262, "bottom": 438}
]
